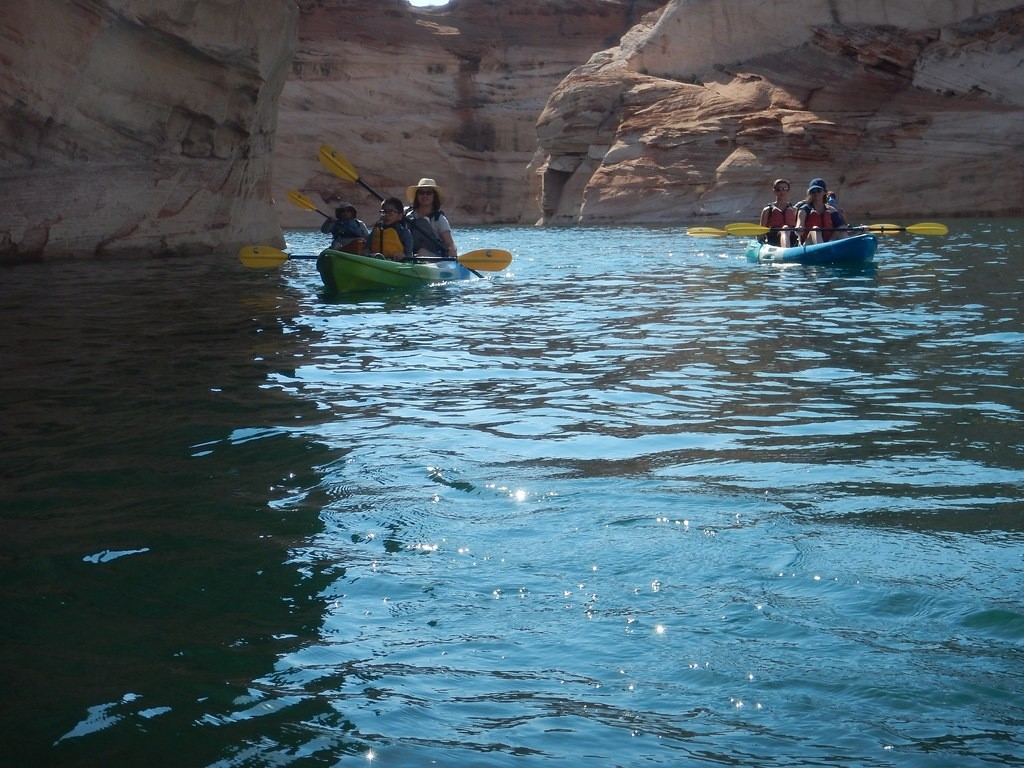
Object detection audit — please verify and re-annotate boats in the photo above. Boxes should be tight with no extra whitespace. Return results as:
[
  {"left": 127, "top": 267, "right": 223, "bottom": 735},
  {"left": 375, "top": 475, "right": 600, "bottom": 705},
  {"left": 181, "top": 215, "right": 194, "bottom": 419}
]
[
  {"left": 744, "top": 233, "right": 878, "bottom": 273},
  {"left": 315, "top": 248, "right": 470, "bottom": 298}
]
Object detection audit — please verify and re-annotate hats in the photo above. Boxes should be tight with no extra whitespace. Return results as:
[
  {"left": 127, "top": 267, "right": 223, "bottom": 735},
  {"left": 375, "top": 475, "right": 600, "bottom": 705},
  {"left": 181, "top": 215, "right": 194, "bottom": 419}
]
[
  {"left": 407, "top": 178, "right": 446, "bottom": 204},
  {"left": 807, "top": 178, "right": 826, "bottom": 194},
  {"left": 335, "top": 201, "right": 357, "bottom": 218}
]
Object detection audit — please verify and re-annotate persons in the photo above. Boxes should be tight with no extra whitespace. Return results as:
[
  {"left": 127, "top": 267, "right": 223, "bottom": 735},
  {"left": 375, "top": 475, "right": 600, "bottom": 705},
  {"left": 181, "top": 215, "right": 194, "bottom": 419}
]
[
  {"left": 760, "top": 179, "right": 799, "bottom": 248},
  {"left": 403, "top": 178, "right": 457, "bottom": 263},
  {"left": 321, "top": 202, "right": 369, "bottom": 248},
  {"left": 363, "top": 197, "right": 413, "bottom": 259},
  {"left": 827, "top": 192, "right": 848, "bottom": 224},
  {"left": 794, "top": 179, "right": 849, "bottom": 247}
]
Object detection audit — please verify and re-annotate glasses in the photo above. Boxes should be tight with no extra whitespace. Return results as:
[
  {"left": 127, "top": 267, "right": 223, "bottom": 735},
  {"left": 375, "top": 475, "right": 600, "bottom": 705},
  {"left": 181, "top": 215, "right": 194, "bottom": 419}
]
[
  {"left": 417, "top": 190, "right": 434, "bottom": 196},
  {"left": 810, "top": 189, "right": 823, "bottom": 194},
  {"left": 774, "top": 187, "right": 789, "bottom": 192},
  {"left": 379, "top": 209, "right": 400, "bottom": 216}
]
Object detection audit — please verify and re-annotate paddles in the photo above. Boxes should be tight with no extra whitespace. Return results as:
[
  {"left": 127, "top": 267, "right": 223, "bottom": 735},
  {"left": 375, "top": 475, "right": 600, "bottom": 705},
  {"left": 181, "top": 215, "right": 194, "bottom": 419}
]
[
  {"left": 687, "top": 222, "right": 902, "bottom": 240},
  {"left": 317, "top": 143, "right": 486, "bottom": 278},
  {"left": 286, "top": 188, "right": 335, "bottom": 221},
  {"left": 725, "top": 220, "right": 952, "bottom": 240},
  {"left": 238, "top": 245, "right": 515, "bottom": 274}
]
